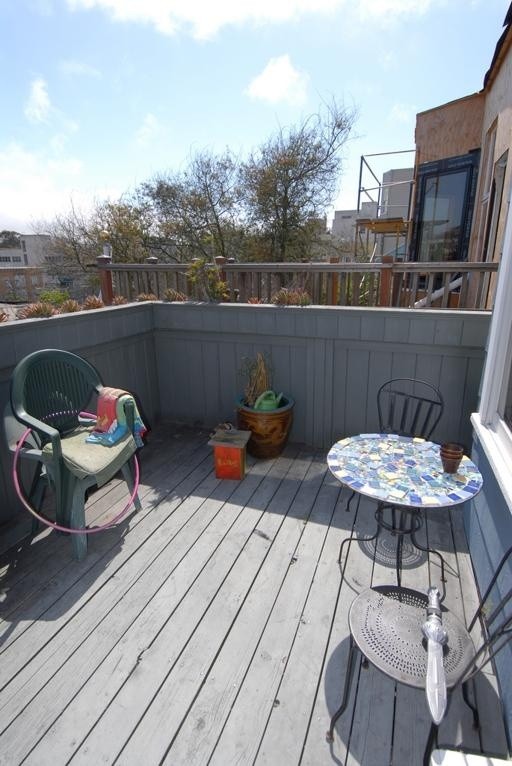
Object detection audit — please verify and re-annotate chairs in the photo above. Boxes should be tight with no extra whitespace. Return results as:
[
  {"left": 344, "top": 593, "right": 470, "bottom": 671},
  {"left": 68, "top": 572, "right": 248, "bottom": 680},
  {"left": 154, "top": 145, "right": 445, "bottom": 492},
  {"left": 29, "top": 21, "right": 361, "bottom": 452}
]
[
  {"left": 327, "top": 546, "right": 512, "bottom": 765},
  {"left": 346, "top": 376, "right": 444, "bottom": 523},
  {"left": 10, "top": 347, "right": 144, "bottom": 559}
]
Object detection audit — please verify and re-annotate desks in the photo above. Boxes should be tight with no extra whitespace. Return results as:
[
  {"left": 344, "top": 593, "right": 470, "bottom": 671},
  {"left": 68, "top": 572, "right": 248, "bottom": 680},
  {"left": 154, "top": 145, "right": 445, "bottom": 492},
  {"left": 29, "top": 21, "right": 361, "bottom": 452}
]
[{"left": 326, "top": 431, "right": 484, "bottom": 602}]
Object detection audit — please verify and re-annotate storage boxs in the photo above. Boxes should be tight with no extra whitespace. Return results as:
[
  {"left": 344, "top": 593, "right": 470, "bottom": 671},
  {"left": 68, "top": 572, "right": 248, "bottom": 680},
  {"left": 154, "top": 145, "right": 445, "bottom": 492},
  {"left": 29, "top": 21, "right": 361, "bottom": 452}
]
[{"left": 204, "top": 425, "right": 251, "bottom": 480}]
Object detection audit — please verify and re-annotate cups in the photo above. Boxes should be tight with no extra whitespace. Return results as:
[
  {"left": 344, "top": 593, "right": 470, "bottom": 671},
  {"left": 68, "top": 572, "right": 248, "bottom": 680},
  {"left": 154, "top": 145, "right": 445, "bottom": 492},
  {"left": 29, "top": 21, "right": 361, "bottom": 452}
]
[{"left": 440, "top": 443, "right": 464, "bottom": 473}]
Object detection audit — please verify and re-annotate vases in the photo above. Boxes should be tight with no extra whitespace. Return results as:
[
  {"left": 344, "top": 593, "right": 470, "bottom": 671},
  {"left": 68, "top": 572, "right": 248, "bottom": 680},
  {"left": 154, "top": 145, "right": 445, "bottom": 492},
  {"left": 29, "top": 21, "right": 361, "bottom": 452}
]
[{"left": 235, "top": 390, "right": 295, "bottom": 459}]
